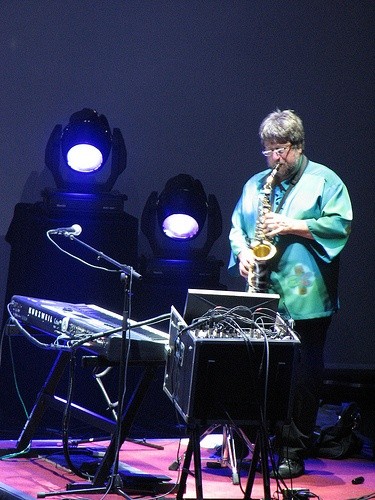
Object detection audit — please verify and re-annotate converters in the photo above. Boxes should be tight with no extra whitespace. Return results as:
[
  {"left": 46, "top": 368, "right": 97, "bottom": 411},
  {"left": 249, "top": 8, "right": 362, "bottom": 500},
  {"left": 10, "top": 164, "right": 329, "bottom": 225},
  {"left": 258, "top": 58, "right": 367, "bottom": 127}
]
[{"left": 282, "top": 488, "right": 310, "bottom": 499}]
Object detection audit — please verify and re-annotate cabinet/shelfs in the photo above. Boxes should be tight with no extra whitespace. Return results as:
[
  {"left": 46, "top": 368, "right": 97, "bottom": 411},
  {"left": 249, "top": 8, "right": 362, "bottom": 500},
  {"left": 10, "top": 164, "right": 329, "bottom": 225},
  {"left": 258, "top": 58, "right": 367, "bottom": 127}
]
[{"left": 0, "top": 202, "right": 227, "bottom": 432}]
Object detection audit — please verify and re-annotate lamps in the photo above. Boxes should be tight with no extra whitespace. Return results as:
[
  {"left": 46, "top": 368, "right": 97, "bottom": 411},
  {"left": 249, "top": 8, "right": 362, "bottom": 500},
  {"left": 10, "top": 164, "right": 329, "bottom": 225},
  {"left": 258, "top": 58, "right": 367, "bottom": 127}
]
[
  {"left": 44, "top": 108, "right": 128, "bottom": 195},
  {"left": 140, "top": 173, "right": 222, "bottom": 262}
]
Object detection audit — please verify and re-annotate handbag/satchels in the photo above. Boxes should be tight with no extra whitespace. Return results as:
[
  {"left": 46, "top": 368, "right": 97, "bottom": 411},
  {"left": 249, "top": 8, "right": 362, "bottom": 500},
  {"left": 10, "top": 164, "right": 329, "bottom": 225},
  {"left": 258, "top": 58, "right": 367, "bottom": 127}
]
[{"left": 315, "top": 404, "right": 362, "bottom": 457}]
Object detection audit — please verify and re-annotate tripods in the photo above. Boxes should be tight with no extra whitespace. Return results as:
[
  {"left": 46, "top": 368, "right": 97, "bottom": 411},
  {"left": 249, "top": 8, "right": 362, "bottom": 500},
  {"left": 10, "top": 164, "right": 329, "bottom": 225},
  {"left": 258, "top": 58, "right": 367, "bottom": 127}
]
[{"left": 37, "top": 234, "right": 158, "bottom": 500}]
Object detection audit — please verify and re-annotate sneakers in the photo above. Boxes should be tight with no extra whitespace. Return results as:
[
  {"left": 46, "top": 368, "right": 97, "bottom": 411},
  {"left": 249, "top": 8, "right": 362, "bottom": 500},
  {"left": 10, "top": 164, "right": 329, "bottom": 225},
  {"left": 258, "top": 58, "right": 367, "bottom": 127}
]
[
  {"left": 209, "top": 440, "right": 248, "bottom": 461},
  {"left": 271, "top": 457, "right": 304, "bottom": 478}
]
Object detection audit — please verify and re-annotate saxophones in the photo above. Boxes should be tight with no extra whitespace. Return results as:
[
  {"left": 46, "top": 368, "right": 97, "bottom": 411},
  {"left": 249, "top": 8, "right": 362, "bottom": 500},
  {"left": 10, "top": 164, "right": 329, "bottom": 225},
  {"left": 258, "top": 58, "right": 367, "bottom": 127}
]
[{"left": 247, "top": 163, "right": 283, "bottom": 295}]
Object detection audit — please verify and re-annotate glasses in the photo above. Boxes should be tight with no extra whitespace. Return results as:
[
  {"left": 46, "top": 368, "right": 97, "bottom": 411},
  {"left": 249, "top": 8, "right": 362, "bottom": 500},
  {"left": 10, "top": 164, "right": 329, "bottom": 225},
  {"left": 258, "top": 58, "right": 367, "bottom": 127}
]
[{"left": 261, "top": 144, "right": 293, "bottom": 157}]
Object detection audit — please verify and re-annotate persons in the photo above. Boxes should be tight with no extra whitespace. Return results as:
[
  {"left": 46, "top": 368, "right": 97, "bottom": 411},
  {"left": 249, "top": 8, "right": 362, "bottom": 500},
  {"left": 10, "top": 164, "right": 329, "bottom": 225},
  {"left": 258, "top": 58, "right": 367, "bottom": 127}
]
[{"left": 206, "top": 109, "right": 352, "bottom": 479}]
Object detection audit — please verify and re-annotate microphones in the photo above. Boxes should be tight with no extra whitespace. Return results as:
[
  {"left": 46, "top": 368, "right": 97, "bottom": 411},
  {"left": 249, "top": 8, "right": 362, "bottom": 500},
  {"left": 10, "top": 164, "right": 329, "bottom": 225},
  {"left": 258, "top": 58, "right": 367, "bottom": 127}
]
[{"left": 49, "top": 224, "right": 82, "bottom": 236}]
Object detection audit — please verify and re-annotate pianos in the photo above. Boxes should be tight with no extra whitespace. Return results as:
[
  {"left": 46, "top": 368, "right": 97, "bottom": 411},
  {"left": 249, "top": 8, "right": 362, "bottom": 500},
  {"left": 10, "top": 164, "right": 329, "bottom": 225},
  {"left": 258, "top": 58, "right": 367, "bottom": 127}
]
[{"left": 1, "top": 295, "right": 186, "bottom": 500}]
